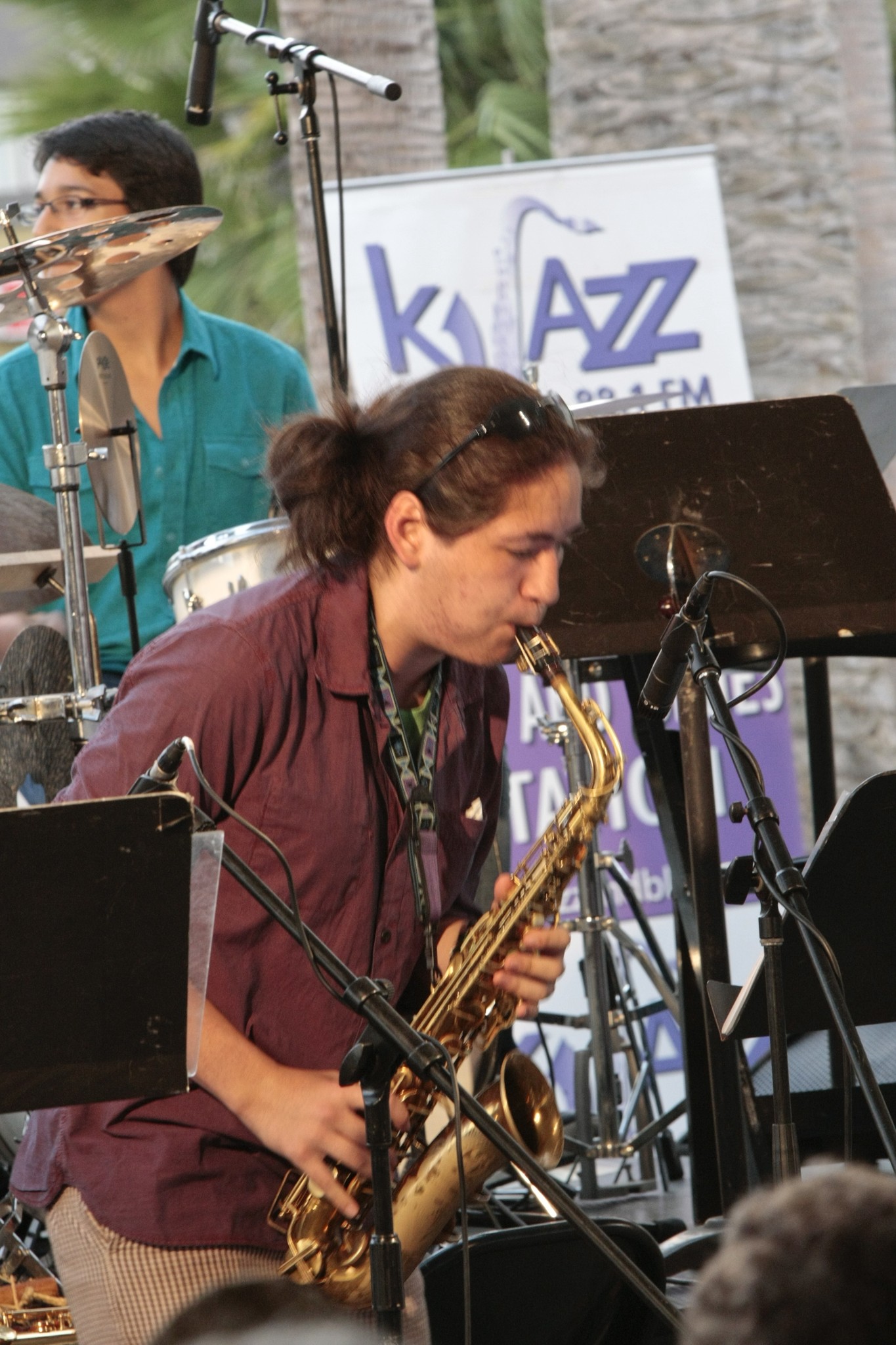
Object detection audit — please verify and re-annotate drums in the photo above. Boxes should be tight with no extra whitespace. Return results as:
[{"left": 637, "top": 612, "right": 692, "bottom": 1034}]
[{"left": 160, "top": 515, "right": 330, "bottom": 624}]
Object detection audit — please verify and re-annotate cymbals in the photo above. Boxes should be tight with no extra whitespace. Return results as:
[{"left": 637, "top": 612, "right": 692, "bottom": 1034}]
[
  {"left": 0, "top": 482, "right": 91, "bottom": 615},
  {"left": 73, "top": 330, "right": 143, "bottom": 536},
  {"left": 0, "top": 625, "right": 83, "bottom": 803},
  {"left": 0, "top": 203, "right": 226, "bottom": 330}
]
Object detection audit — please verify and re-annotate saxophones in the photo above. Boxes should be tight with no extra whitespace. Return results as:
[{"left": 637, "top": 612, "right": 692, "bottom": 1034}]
[{"left": 268, "top": 621, "right": 630, "bottom": 1315}]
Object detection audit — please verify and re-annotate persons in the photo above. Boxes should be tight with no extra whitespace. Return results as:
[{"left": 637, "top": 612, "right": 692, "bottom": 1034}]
[
  {"left": 0, "top": 109, "right": 322, "bottom": 687},
  {"left": 10, "top": 365, "right": 608, "bottom": 1345}
]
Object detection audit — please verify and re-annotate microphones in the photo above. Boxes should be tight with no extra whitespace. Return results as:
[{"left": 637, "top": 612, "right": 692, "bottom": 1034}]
[
  {"left": 184, "top": 0, "right": 223, "bottom": 127},
  {"left": 636, "top": 570, "right": 715, "bottom": 724},
  {"left": 124, "top": 736, "right": 188, "bottom": 797}
]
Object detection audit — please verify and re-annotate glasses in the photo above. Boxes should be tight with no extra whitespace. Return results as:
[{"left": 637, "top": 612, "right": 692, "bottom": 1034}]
[
  {"left": 413, "top": 390, "right": 579, "bottom": 498},
  {"left": 17, "top": 194, "right": 130, "bottom": 228}
]
